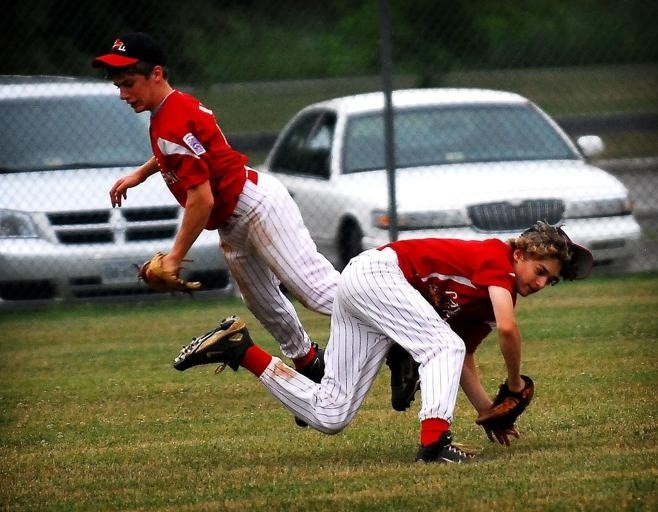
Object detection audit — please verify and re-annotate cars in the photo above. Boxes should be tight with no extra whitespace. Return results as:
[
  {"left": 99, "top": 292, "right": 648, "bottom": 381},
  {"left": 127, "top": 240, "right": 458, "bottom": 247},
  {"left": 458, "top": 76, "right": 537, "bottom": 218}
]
[
  {"left": 243, "top": 85, "right": 644, "bottom": 276},
  {"left": 0, "top": 70, "right": 239, "bottom": 305}
]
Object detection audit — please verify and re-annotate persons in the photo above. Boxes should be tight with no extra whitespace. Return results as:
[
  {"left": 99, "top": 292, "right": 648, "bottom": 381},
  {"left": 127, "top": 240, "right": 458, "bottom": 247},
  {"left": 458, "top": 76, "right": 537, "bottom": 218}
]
[
  {"left": 174, "top": 221, "right": 595, "bottom": 463},
  {"left": 89, "top": 32, "right": 422, "bottom": 428}
]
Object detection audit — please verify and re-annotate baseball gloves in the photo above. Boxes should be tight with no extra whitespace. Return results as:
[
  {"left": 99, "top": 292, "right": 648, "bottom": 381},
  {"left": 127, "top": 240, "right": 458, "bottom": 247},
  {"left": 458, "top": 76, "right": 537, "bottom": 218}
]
[
  {"left": 131, "top": 250, "right": 202, "bottom": 293},
  {"left": 475, "top": 375, "right": 534, "bottom": 430}
]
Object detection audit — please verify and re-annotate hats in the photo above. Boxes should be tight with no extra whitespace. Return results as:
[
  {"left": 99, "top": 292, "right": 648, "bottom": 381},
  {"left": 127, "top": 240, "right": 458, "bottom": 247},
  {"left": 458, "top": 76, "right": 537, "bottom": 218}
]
[
  {"left": 521, "top": 226, "right": 594, "bottom": 280},
  {"left": 92, "top": 31, "right": 167, "bottom": 67}
]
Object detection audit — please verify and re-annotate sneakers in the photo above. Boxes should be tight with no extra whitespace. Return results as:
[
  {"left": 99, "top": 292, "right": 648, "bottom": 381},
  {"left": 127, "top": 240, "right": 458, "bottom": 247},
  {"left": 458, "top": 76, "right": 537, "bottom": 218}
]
[
  {"left": 415, "top": 430, "right": 476, "bottom": 463},
  {"left": 172, "top": 314, "right": 253, "bottom": 374},
  {"left": 294, "top": 342, "right": 325, "bottom": 427},
  {"left": 385, "top": 343, "right": 421, "bottom": 411}
]
